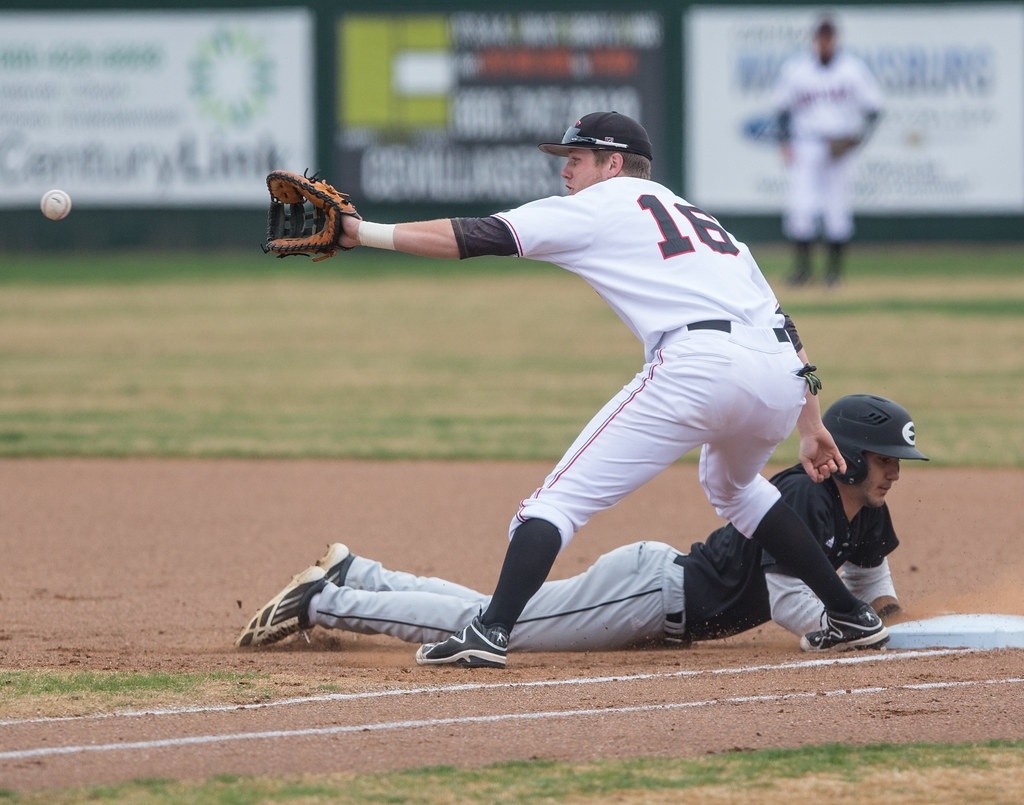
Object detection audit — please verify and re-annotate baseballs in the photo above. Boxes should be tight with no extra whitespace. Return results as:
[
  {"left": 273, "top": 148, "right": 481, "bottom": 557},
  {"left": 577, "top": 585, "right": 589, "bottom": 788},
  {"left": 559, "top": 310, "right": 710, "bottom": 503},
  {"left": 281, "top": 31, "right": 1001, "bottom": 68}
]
[{"left": 40, "top": 190, "right": 73, "bottom": 221}]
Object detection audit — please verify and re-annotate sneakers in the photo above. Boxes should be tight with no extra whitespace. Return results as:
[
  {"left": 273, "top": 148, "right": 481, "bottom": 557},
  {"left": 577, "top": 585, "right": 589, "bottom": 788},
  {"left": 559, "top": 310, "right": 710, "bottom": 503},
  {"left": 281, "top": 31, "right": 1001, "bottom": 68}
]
[
  {"left": 414, "top": 604, "right": 510, "bottom": 667},
  {"left": 799, "top": 599, "right": 891, "bottom": 652},
  {"left": 233, "top": 566, "right": 329, "bottom": 649},
  {"left": 314, "top": 542, "right": 355, "bottom": 583}
]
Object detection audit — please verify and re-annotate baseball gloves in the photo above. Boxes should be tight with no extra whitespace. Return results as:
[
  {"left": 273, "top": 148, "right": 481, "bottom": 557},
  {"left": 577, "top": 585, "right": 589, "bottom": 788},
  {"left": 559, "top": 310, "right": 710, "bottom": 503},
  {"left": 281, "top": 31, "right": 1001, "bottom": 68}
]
[
  {"left": 825, "top": 134, "right": 859, "bottom": 157},
  {"left": 258, "top": 166, "right": 364, "bottom": 263}
]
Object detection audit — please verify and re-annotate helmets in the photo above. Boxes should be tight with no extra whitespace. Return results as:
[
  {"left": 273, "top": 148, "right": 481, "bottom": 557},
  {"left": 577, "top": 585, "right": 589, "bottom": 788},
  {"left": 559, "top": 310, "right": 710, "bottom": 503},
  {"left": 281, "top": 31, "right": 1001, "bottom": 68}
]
[{"left": 821, "top": 394, "right": 930, "bottom": 485}]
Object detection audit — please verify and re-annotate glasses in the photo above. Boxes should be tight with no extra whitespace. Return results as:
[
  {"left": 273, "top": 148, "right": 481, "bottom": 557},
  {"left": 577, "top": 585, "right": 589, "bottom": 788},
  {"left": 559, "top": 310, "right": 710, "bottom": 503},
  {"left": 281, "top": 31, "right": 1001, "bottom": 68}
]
[{"left": 562, "top": 127, "right": 629, "bottom": 148}]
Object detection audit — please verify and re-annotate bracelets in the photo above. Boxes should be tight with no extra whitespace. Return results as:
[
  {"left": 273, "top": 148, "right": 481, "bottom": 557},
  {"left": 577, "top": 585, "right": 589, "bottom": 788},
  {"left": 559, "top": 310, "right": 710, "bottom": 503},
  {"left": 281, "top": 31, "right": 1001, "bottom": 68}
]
[{"left": 877, "top": 604, "right": 901, "bottom": 618}]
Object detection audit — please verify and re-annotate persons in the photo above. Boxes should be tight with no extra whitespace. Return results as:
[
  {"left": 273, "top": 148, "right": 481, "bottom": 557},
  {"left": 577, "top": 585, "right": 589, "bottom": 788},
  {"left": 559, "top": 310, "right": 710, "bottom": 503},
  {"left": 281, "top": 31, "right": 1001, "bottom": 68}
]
[
  {"left": 774, "top": 15, "right": 883, "bottom": 284},
  {"left": 260, "top": 111, "right": 892, "bottom": 668},
  {"left": 232, "top": 394, "right": 929, "bottom": 645}
]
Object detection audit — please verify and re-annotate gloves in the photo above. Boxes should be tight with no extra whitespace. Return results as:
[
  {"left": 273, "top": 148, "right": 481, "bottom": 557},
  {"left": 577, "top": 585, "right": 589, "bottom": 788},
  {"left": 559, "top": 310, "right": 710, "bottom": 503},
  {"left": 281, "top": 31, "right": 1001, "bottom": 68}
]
[{"left": 796, "top": 362, "right": 821, "bottom": 396}]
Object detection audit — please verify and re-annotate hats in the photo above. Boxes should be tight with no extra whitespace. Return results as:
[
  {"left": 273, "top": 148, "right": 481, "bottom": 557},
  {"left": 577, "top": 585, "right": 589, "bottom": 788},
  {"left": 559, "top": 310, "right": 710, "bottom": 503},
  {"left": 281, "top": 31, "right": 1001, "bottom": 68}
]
[{"left": 537, "top": 110, "right": 653, "bottom": 161}]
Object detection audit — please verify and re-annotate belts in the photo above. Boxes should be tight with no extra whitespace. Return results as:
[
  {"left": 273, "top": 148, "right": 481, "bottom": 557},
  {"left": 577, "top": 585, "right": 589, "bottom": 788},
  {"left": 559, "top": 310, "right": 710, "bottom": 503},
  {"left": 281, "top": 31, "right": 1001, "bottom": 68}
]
[
  {"left": 686, "top": 320, "right": 790, "bottom": 342},
  {"left": 667, "top": 555, "right": 688, "bottom": 640}
]
